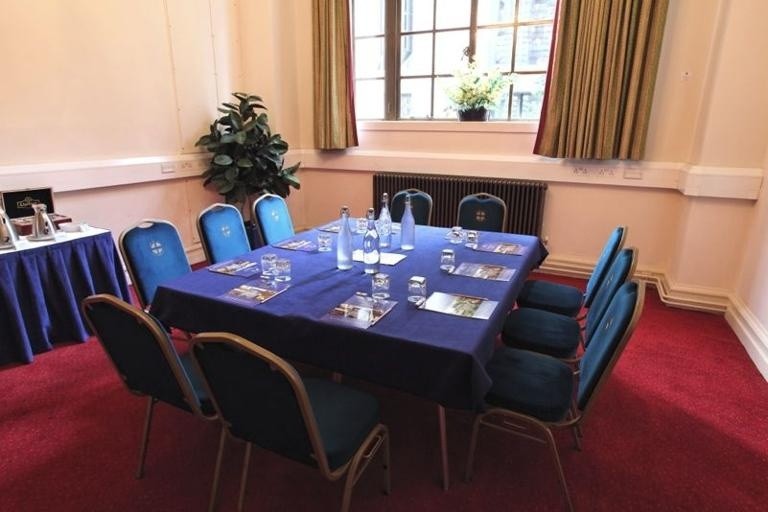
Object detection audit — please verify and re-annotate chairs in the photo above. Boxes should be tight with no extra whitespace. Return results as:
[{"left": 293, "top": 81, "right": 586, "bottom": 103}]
[
  {"left": 463, "top": 279, "right": 645, "bottom": 512},
  {"left": 196, "top": 203, "right": 252, "bottom": 267},
  {"left": 118, "top": 217, "right": 193, "bottom": 311},
  {"left": 252, "top": 194, "right": 296, "bottom": 245},
  {"left": 515, "top": 225, "right": 628, "bottom": 322},
  {"left": 190, "top": 333, "right": 390, "bottom": 512},
  {"left": 457, "top": 193, "right": 507, "bottom": 233},
  {"left": 501, "top": 246, "right": 639, "bottom": 364},
  {"left": 82, "top": 294, "right": 228, "bottom": 511},
  {"left": 390, "top": 188, "right": 433, "bottom": 226}
]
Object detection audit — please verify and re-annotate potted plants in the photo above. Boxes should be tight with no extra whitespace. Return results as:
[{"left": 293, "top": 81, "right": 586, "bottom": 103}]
[
  {"left": 445, "top": 54, "right": 518, "bottom": 122},
  {"left": 194, "top": 92, "right": 302, "bottom": 251}
]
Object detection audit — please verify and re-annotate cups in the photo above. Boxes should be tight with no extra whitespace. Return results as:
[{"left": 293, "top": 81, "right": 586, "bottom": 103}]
[
  {"left": 261, "top": 254, "right": 275, "bottom": 278},
  {"left": 276, "top": 260, "right": 291, "bottom": 282},
  {"left": 440, "top": 249, "right": 456, "bottom": 272},
  {"left": 450, "top": 226, "right": 462, "bottom": 244},
  {"left": 355, "top": 217, "right": 368, "bottom": 234},
  {"left": 372, "top": 273, "right": 390, "bottom": 298},
  {"left": 467, "top": 230, "right": 479, "bottom": 249},
  {"left": 408, "top": 275, "right": 427, "bottom": 303},
  {"left": 317, "top": 233, "right": 332, "bottom": 252}
]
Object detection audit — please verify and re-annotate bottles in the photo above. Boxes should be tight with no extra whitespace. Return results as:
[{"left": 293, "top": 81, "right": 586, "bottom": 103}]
[
  {"left": 336, "top": 206, "right": 352, "bottom": 270},
  {"left": 400, "top": 195, "right": 414, "bottom": 250},
  {"left": 377, "top": 193, "right": 392, "bottom": 248},
  {"left": 362, "top": 208, "right": 379, "bottom": 273}
]
